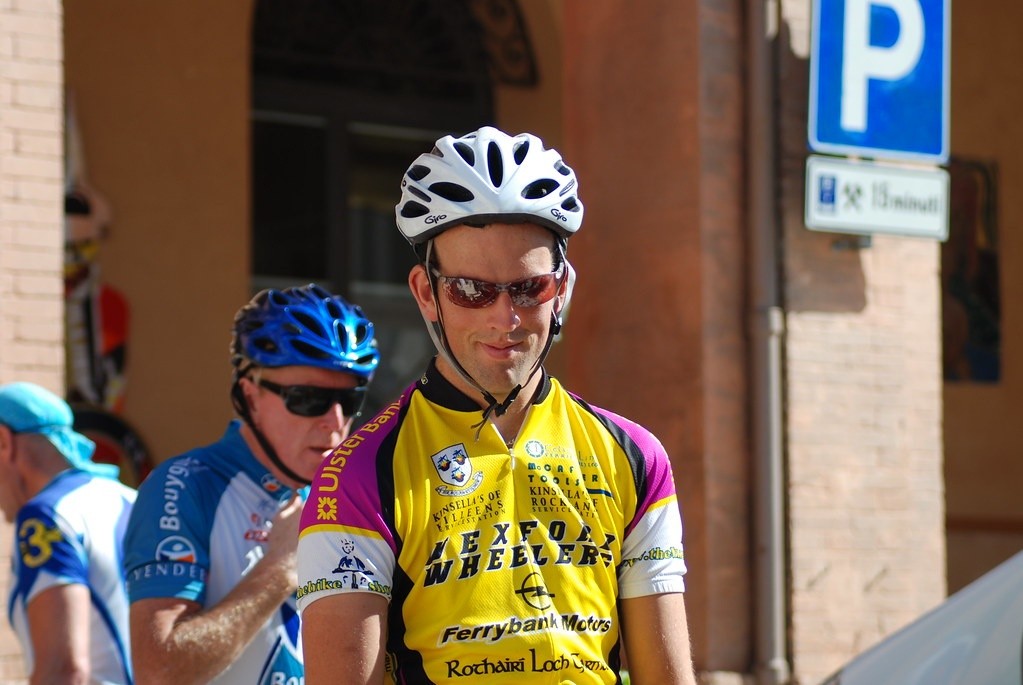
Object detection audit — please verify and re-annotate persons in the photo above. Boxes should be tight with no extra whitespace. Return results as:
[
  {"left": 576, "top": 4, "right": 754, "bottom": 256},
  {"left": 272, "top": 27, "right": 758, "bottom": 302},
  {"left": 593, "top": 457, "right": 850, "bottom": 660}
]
[
  {"left": 0, "top": 380, "right": 139, "bottom": 685},
  {"left": 121, "top": 280, "right": 382, "bottom": 685},
  {"left": 296, "top": 124, "right": 697, "bottom": 685}
]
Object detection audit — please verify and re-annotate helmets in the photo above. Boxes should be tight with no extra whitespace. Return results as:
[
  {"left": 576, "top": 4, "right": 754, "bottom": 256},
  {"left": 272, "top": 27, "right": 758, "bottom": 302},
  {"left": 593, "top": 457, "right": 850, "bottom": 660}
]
[
  {"left": 393, "top": 126, "right": 585, "bottom": 239},
  {"left": 230, "top": 285, "right": 380, "bottom": 385}
]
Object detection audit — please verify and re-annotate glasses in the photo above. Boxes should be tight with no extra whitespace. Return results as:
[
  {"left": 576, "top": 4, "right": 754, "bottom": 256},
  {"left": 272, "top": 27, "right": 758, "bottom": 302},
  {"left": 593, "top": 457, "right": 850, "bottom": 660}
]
[
  {"left": 424, "top": 238, "right": 570, "bottom": 308},
  {"left": 249, "top": 374, "right": 368, "bottom": 418}
]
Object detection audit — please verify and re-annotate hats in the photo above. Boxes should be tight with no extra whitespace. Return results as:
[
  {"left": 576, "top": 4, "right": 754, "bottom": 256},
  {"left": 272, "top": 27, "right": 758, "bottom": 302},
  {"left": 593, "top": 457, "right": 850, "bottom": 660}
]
[{"left": 0, "top": 381, "right": 121, "bottom": 480}]
